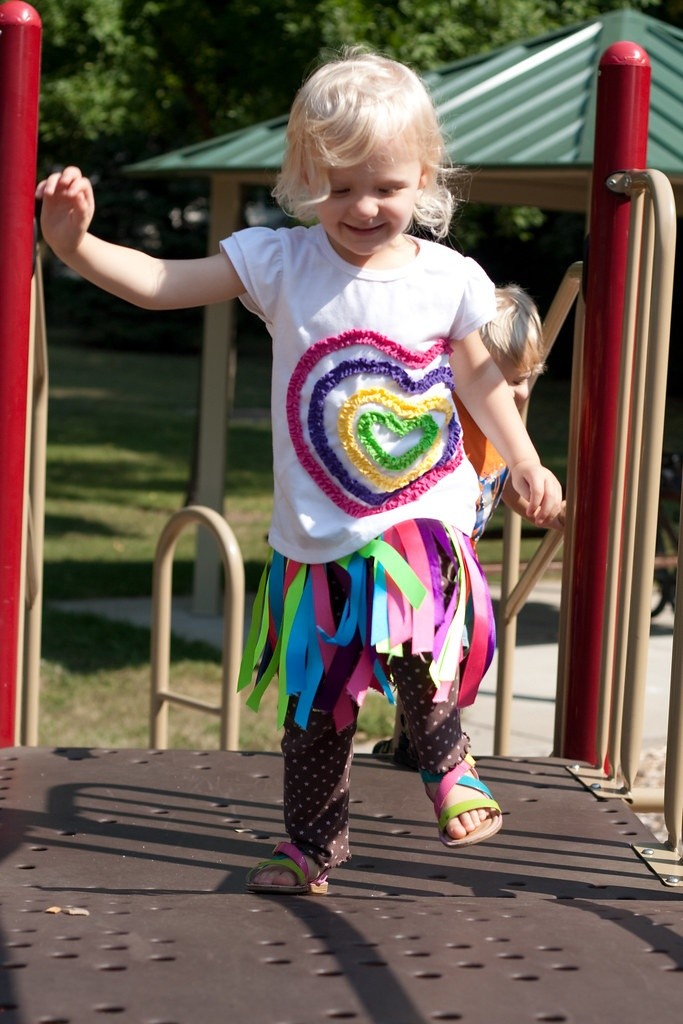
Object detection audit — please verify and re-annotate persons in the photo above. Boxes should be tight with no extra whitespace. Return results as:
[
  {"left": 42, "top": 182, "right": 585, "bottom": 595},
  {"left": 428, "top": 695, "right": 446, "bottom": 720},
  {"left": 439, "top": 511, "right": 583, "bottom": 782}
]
[
  {"left": 35, "top": 49, "right": 563, "bottom": 893},
  {"left": 372, "top": 284, "right": 570, "bottom": 777}
]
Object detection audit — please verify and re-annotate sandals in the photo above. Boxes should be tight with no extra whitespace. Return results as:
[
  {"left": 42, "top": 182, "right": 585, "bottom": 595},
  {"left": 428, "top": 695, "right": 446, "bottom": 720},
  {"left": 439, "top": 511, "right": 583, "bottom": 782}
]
[
  {"left": 420, "top": 752, "right": 503, "bottom": 848},
  {"left": 244, "top": 840, "right": 329, "bottom": 892}
]
